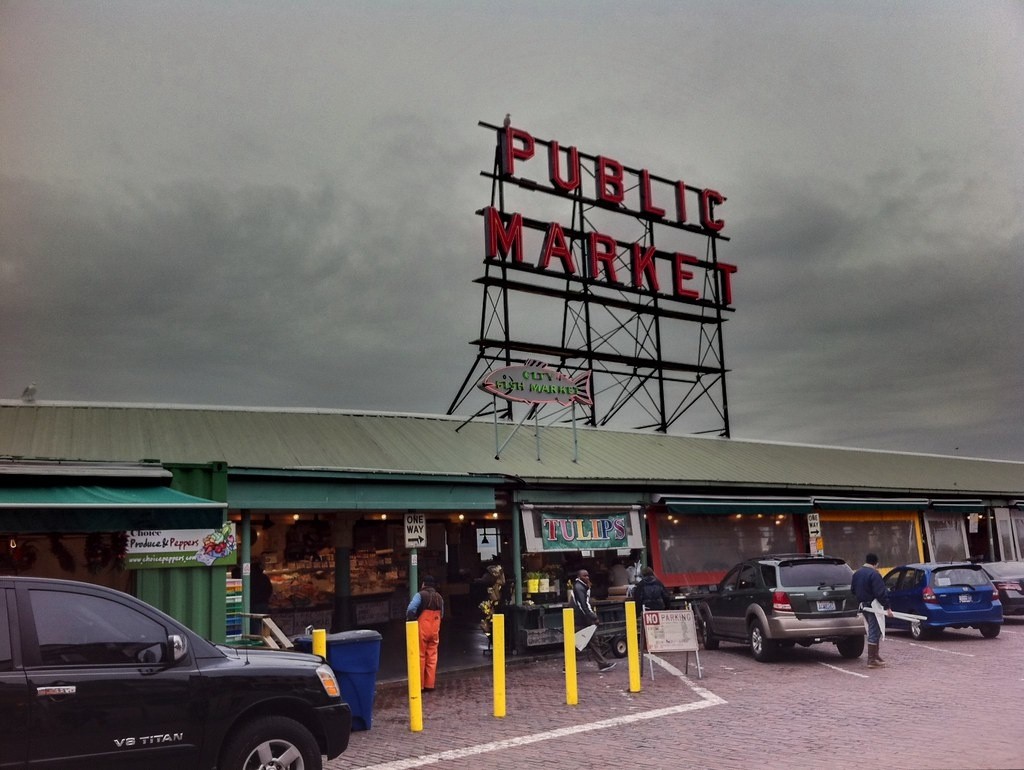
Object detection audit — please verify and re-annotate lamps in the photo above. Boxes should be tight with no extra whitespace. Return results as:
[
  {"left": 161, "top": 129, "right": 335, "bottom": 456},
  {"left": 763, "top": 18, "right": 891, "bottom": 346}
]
[
  {"left": 263, "top": 515, "right": 275, "bottom": 530},
  {"left": 480, "top": 528, "right": 489, "bottom": 544}
]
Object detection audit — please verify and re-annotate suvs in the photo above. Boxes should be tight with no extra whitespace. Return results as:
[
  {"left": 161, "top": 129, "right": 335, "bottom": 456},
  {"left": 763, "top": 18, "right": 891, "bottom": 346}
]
[{"left": 699, "top": 553, "right": 868, "bottom": 660}]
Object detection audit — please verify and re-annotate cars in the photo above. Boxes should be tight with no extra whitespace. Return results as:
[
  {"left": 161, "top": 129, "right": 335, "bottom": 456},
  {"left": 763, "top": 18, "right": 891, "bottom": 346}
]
[
  {"left": 976, "top": 562, "right": 1024, "bottom": 616},
  {"left": 0, "top": 575, "right": 351, "bottom": 769},
  {"left": 883, "top": 562, "right": 1003, "bottom": 641}
]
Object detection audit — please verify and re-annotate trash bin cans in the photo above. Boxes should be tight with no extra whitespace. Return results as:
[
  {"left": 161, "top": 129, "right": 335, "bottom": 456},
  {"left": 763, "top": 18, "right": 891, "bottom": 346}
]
[
  {"left": 295, "top": 627, "right": 384, "bottom": 732},
  {"left": 226, "top": 638, "right": 264, "bottom": 647}
]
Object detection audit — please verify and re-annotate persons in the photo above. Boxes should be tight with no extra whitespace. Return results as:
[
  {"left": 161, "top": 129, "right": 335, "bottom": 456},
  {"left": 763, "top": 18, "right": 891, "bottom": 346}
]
[
  {"left": 634, "top": 567, "right": 671, "bottom": 618},
  {"left": 473, "top": 557, "right": 499, "bottom": 600},
  {"left": 851, "top": 553, "right": 894, "bottom": 667},
  {"left": 231, "top": 560, "right": 273, "bottom": 634},
  {"left": 562, "top": 569, "right": 617, "bottom": 674},
  {"left": 559, "top": 552, "right": 592, "bottom": 581},
  {"left": 607, "top": 558, "right": 629, "bottom": 586},
  {"left": 406, "top": 574, "right": 444, "bottom": 692}
]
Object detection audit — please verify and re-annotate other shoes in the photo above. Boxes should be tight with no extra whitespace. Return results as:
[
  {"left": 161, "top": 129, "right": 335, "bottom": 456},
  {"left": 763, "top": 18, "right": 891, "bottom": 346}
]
[
  {"left": 563, "top": 669, "right": 580, "bottom": 674},
  {"left": 422, "top": 688, "right": 435, "bottom": 692},
  {"left": 600, "top": 662, "right": 617, "bottom": 671}
]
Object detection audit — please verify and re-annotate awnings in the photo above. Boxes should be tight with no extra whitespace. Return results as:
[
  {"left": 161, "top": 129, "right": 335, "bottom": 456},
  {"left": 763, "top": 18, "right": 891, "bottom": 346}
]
[
  {"left": 0, "top": 463, "right": 229, "bottom": 533},
  {"left": 1008, "top": 499, "right": 1024, "bottom": 511},
  {"left": 814, "top": 496, "right": 929, "bottom": 511},
  {"left": 929, "top": 498, "right": 985, "bottom": 512},
  {"left": 652, "top": 493, "right": 814, "bottom": 514}
]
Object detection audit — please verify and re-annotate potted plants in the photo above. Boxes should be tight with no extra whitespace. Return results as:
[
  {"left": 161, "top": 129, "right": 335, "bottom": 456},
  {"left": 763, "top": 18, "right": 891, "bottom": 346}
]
[{"left": 523, "top": 572, "right": 551, "bottom": 593}]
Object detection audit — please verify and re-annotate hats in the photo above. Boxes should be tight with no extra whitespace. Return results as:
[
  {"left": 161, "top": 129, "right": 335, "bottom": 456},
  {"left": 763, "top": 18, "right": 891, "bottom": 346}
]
[
  {"left": 866, "top": 553, "right": 879, "bottom": 563},
  {"left": 421, "top": 575, "right": 433, "bottom": 582}
]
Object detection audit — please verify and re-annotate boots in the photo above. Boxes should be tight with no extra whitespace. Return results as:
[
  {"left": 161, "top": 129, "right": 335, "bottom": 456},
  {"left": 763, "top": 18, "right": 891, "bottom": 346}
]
[{"left": 866, "top": 642, "right": 886, "bottom": 667}]
